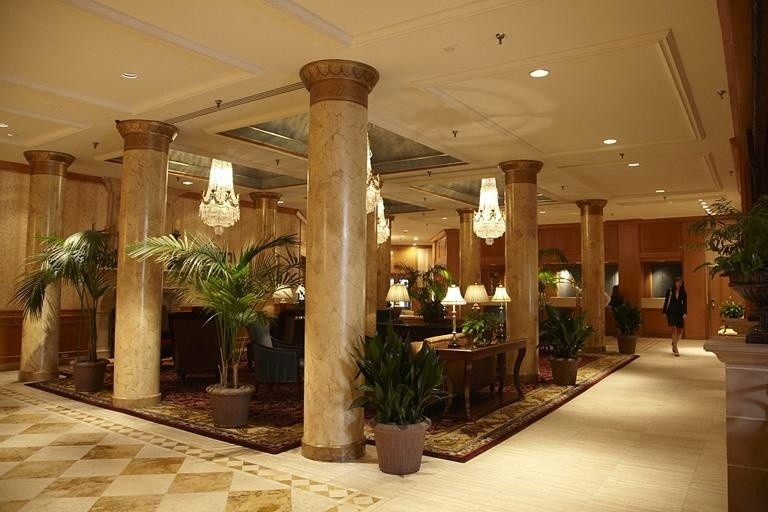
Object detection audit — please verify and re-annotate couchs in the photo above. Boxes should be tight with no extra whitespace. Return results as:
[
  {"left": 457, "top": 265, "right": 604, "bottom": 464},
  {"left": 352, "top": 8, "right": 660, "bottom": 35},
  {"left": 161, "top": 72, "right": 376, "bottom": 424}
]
[
  {"left": 168, "top": 305, "right": 232, "bottom": 384},
  {"left": 246, "top": 308, "right": 305, "bottom": 396}
]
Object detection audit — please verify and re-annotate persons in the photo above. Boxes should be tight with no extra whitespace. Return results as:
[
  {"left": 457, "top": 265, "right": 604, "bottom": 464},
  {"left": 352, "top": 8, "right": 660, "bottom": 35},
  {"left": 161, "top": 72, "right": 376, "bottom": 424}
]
[{"left": 663, "top": 277, "right": 687, "bottom": 357}]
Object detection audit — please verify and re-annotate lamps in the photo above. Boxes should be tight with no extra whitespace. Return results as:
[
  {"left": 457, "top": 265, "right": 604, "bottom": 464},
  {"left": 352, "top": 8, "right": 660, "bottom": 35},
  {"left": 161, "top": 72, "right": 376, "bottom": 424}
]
[
  {"left": 366, "top": 131, "right": 383, "bottom": 217},
  {"left": 197, "top": 157, "right": 242, "bottom": 238},
  {"left": 472, "top": 176, "right": 506, "bottom": 246},
  {"left": 271, "top": 280, "right": 512, "bottom": 348},
  {"left": 377, "top": 194, "right": 391, "bottom": 245}
]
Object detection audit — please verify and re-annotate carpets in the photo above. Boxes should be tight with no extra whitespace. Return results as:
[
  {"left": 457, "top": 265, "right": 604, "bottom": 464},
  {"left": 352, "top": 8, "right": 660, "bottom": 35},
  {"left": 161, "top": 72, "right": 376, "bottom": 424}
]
[{"left": 25, "top": 348, "right": 642, "bottom": 467}]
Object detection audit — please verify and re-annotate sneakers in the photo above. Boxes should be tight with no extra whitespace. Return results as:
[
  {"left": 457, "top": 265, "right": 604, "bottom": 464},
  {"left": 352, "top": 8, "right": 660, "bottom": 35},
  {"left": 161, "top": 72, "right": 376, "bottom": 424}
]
[{"left": 672, "top": 347, "right": 681, "bottom": 357}]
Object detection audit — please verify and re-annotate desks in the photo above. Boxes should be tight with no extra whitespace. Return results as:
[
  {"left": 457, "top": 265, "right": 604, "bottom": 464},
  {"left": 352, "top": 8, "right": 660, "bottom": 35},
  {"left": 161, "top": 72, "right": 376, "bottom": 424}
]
[{"left": 433, "top": 334, "right": 530, "bottom": 419}]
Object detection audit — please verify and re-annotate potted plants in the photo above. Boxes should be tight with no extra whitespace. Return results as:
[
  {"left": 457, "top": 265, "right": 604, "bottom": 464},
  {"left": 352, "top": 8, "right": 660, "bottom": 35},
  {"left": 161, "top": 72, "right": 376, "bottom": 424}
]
[
  {"left": 347, "top": 310, "right": 458, "bottom": 477},
  {"left": 2, "top": 220, "right": 118, "bottom": 396},
  {"left": 674, "top": 193, "right": 768, "bottom": 347},
  {"left": 536, "top": 303, "right": 598, "bottom": 388},
  {"left": 407, "top": 264, "right": 449, "bottom": 323},
  {"left": 610, "top": 295, "right": 645, "bottom": 355},
  {"left": 123, "top": 231, "right": 306, "bottom": 431}
]
